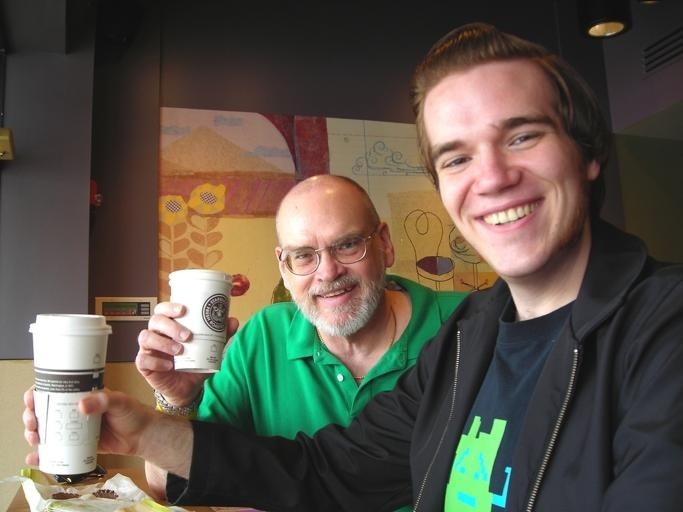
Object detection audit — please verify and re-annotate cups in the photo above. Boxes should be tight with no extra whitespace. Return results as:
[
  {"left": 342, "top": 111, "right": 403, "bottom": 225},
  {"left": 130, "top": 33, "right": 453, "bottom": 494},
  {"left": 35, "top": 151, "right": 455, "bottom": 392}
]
[
  {"left": 28, "top": 314, "right": 112, "bottom": 476},
  {"left": 167, "top": 270, "right": 233, "bottom": 371}
]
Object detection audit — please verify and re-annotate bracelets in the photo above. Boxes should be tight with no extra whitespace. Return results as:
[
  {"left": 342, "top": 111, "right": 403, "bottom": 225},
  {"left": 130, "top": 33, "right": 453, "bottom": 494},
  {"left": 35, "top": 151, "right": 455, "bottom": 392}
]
[{"left": 154, "top": 385, "right": 204, "bottom": 416}]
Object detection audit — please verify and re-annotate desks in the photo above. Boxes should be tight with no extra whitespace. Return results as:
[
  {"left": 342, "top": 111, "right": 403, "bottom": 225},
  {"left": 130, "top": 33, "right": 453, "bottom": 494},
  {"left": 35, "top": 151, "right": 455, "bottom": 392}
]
[{"left": 3, "top": 465, "right": 216, "bottom": 512}]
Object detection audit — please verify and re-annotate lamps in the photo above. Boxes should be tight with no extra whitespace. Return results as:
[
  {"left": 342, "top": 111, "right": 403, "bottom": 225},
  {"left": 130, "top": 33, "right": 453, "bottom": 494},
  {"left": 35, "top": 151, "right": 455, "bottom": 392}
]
[{"left": 577, "top": 0, "right": 632, "bottom": 41}]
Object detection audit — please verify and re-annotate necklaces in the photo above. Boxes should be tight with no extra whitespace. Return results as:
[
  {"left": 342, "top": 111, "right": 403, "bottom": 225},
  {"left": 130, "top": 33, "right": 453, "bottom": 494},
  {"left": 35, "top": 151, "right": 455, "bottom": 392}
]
[{"left": 317, "top": 306, "right": 397, "bottom": 379}]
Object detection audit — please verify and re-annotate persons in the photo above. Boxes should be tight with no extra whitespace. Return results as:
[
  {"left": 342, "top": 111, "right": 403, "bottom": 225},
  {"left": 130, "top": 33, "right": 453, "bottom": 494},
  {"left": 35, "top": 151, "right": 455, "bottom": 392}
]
[
  {"left": 23, "top": 24, "right": 682, "bottom": 511},
  {"left": 136, "top": 174, "right": 470, "bottom": 499}
]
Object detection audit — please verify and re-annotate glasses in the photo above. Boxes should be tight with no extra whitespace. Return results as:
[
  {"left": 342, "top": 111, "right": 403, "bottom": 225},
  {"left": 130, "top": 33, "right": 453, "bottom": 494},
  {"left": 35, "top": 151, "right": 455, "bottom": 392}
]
[{"left": 279, "top": 230, "right": 376, "bottom": 276}]
